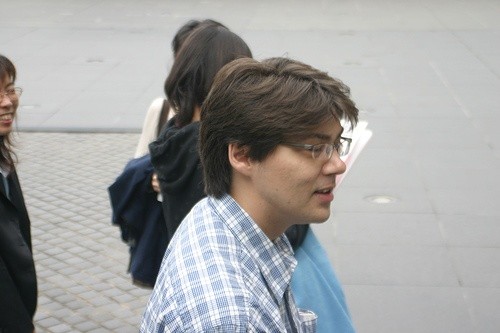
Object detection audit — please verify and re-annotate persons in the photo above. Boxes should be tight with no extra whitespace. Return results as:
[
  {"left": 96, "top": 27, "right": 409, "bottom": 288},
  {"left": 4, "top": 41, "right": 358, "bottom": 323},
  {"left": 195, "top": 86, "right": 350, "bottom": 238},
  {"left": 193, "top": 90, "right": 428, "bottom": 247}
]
[
  {"left": 148, "top": 25, "right": 311, "bottom": 253},
  {"left": 138, "top": 58, "right": 359, "bottom": 333},
  {"left": 0, "top": 54, "right": 38, "bottom": 333},
  {"left": 134, "top": 18, "right": 223, "bottom": 191}
]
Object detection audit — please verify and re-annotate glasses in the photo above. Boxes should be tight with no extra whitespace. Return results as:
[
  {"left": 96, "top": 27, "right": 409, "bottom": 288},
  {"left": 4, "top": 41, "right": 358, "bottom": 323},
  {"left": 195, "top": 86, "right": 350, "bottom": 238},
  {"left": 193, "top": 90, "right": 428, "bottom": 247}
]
[
  {"left": 0, "top": 87, "right": 23, "bottom": 101},
  {"left": 279, "top": 137, "right": 352, "bottom": 164}
]
